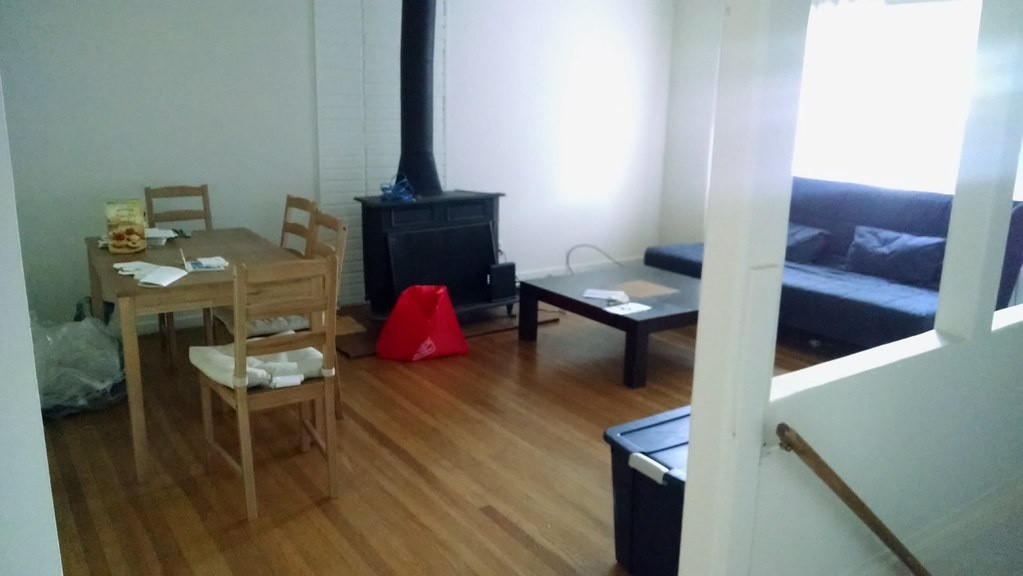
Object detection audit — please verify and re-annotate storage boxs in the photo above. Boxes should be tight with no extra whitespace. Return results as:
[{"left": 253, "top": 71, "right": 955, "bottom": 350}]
[{"left": 602, "top": 404, "right": 692, "bottom": 576}]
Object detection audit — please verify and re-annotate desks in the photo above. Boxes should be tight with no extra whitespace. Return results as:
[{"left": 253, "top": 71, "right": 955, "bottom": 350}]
[
  {"left": 518, "top": 265, "right": 700, "bottom": 390},
  {"left": 84, "top": 226, "right": 302, "bottom": 485}
]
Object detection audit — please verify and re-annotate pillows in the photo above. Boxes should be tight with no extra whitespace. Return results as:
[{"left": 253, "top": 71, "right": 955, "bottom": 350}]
[
  {"left": 838, "top": 223, "right": 946, "bottom": 290},
  {"left": 785, "top": 222, "right": 832, "bottom": 266}
]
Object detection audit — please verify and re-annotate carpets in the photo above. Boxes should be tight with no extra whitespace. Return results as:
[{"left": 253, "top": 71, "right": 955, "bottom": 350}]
[{"left": 335, "top": 304, "right": 560, "bottom": 361}]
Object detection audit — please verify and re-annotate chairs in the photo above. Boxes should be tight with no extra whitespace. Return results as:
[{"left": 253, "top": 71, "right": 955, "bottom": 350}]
[
  {"left": 144, "top": 183, "right": 212, "bottom": 373},
  {"left": 212, "top": 207, "right": 349, "bottom": 437},
  {"left": 188, "top": 253, "right": 340, "bottom": 522},
  {"left": 279, "top": 193, "right": 318, "bottom": 258}
]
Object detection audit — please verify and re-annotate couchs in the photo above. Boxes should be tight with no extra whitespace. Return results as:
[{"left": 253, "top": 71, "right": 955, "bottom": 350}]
[{"left": 644, "top": 174, "right": 1023, "bottom": 348}]
